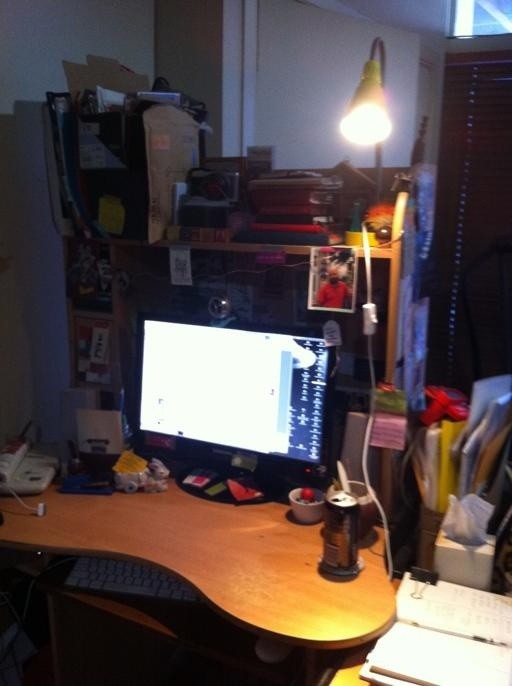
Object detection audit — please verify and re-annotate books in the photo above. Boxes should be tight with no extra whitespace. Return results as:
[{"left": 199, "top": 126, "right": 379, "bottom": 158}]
[
  {"left": 424, "top": 374, "right": 512, "bottom": 512},
  {"left": 230, "top": 177, "right": 343, "bottom": 245},
  {"left": 359, "top": 571, "right": 512, "bottom": 686}
]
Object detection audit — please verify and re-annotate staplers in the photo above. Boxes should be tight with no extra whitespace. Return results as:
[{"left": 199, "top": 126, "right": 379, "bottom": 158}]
[{"left": 60, "top": 472, "right": 113, "bottom": 494}]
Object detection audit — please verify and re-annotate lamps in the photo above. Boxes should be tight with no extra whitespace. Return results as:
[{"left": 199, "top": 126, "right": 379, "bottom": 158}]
[{"left": 333, "top": 36, "right": 397, "bottom": 251}]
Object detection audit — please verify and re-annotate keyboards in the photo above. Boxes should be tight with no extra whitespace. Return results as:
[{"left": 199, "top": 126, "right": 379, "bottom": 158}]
[{"left": 64, "top": 557, "right": 199, "bottom": 602}]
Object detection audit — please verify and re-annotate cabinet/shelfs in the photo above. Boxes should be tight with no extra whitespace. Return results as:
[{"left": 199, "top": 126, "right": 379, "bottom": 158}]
[{"left": 59, "top": 236, "right": 441, "bottom": 524}]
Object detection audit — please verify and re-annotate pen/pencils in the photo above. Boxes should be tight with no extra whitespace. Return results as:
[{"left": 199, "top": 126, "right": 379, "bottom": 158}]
[{"left": 413, "top": 621, "right": 508, "bottom": 647}]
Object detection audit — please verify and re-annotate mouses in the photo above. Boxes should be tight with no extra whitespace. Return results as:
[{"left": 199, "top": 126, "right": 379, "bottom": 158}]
[{"left": 255, "top": 638, "right": 293, "bottom": 664}]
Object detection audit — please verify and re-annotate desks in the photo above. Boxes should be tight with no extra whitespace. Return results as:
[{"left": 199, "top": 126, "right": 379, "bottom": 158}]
[
  {"left": 0, "top": 435, "right": 400, "bottom": 686},
  {"left": 311, "top": 562, "right": 512, "bottom": 686}
]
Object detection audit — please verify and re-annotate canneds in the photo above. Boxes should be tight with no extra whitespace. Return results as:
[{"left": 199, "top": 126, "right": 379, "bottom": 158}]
[{"left": 322, "top": 495, "right": 357, "bottom": 577}]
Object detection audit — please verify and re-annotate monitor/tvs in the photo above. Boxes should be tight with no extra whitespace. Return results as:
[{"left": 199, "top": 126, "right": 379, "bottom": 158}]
[{"left": 133, "top": 311, "right": 336, "bottom": 505}]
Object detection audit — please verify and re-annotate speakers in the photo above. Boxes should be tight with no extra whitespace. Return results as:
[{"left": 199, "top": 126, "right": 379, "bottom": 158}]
[{"left": 340, "top": 411, "right": 419, "bottom": 512}]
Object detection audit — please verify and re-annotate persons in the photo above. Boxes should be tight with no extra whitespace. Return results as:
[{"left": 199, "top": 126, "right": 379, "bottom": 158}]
[{"left": 317, "top": 265, "right": 352, "bottom": 308}]
